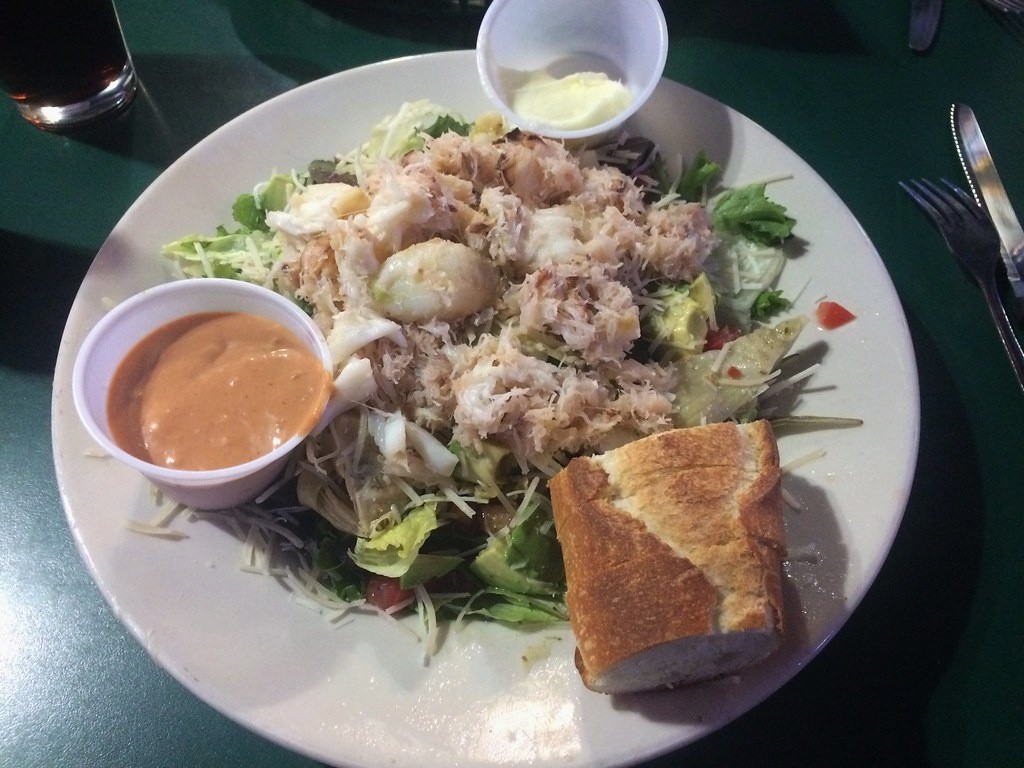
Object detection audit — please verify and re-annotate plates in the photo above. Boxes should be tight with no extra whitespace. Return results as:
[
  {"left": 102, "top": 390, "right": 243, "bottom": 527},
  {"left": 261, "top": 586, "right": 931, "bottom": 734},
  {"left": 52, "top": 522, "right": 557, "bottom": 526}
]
[{"left": 50, "top": 48, "right": 920, "bottom": 768}]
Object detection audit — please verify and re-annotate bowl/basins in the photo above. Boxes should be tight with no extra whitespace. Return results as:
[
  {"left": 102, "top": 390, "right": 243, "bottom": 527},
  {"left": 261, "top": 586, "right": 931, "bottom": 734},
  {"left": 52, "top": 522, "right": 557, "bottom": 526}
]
[
  {"left": 477, "top": 0, "right": 671, "bottom": 149},
  {"left": 70, "top": 276, "right": 333, "bottom": 512}
]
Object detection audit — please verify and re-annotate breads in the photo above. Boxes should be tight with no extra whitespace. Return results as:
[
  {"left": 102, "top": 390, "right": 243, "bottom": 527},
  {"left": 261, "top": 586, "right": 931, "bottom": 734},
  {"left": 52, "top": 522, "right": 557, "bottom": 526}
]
[{"left": 549, "top": 419, "right": 791, "bottom": 695}]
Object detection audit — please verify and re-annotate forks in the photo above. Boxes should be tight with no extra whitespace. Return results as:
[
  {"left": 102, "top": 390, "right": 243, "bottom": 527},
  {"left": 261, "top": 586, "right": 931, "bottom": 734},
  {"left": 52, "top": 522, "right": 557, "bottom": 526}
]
[{"left": 899, "top": 177, "right": 1023, "bottom": 395}]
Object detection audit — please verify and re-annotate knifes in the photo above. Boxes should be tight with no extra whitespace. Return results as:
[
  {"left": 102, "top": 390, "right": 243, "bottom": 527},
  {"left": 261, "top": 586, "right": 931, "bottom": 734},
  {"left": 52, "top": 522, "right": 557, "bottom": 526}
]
[{"left": 950, "top": 104, "right": 1024, "bottom": 298}]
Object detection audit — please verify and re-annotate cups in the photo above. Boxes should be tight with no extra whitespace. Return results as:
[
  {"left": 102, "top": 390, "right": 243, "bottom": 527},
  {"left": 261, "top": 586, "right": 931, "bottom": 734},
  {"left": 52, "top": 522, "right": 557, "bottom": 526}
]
[{"left": 0, "top": 0, "right": 139, "bottom": 130}]
